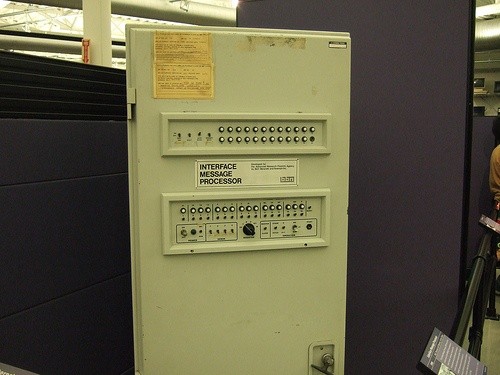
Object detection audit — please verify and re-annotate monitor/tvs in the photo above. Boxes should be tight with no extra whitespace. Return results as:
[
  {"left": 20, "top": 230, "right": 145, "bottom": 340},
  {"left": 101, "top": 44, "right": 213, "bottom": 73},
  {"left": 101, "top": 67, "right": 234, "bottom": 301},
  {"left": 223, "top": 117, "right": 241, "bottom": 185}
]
[
  {"left": 494, "top": 81, "right": 500, "bottom": 93},
  {"left": 474, "top": 77, "right": 485, "bottom": 88}
]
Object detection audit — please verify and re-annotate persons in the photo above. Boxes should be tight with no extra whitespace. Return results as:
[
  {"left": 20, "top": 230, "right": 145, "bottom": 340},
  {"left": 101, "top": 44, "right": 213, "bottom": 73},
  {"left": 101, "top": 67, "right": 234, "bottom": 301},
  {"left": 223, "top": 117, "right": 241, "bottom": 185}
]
[{"left": 489, "top": 141, "right": 500, "bottom": 226}]
